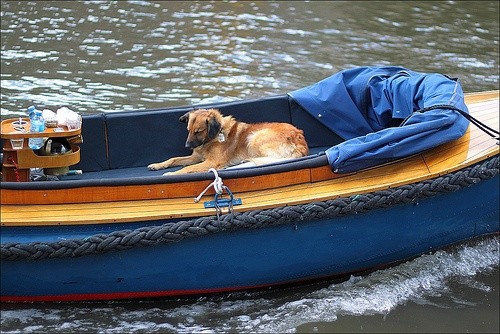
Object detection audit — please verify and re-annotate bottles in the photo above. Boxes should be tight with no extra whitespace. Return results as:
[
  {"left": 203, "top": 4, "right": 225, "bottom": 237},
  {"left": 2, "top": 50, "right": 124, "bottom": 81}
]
[
  {"left": 28, "top": 106, "right": 50, "bottom": 141},
  {"left": 29, "top": 111, "right": 47, "bottom": 150}
]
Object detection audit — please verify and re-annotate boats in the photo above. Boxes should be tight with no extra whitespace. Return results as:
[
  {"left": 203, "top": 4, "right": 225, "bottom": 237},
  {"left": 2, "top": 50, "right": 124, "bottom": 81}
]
[{"left": 0, "top": 88, "right": 500, "bottom": 303}]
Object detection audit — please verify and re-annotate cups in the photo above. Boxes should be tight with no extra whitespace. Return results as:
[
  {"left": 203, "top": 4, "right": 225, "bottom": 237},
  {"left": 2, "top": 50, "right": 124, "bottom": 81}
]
[{"left": 10, "top": 138, "right": 24, "bottom": 149}]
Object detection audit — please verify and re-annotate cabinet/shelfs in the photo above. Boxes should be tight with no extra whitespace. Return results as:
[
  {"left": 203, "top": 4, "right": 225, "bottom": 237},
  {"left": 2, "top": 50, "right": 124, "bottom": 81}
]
[{"left": 0, "top": 118, "right": 83, "bottom": 183}]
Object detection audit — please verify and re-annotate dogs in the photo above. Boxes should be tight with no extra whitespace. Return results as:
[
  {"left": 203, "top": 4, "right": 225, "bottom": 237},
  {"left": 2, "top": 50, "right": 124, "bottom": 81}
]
[{"left": 146, "top": 108, "right": 309, "bottom": 176}]
